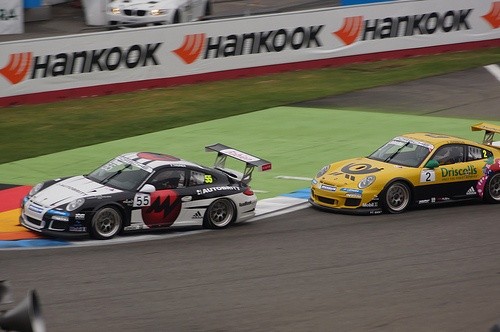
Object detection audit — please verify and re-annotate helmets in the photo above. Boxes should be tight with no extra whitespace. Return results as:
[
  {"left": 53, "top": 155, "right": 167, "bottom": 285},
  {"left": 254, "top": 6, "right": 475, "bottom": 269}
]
[
  {"left": 436, "top": 147, "right": 450, "bottom": 160},
  {"left": 156, "top": 172, "right": 180, "bottom": 190}
]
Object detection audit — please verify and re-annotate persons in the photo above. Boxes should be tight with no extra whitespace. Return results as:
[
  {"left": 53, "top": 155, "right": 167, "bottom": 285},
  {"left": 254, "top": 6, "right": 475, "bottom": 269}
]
[{"left": 436, "top": 148, "right": 461, "bottom": 164}]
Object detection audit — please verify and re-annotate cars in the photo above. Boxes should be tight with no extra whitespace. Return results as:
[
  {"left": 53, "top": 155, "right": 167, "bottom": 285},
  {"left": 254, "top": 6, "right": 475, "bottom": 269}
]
[{"left": 105, "top": 0, "right": 216, "bottom": 30}]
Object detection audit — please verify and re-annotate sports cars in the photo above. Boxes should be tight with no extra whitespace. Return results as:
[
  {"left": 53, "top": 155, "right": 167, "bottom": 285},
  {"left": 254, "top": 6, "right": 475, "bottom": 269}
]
[
  {"left": 309, "top": 121, "right": 499, "bottom": 213},
  {"left": 20, "top": 140, "right": 273, "bottom": 240}
]
